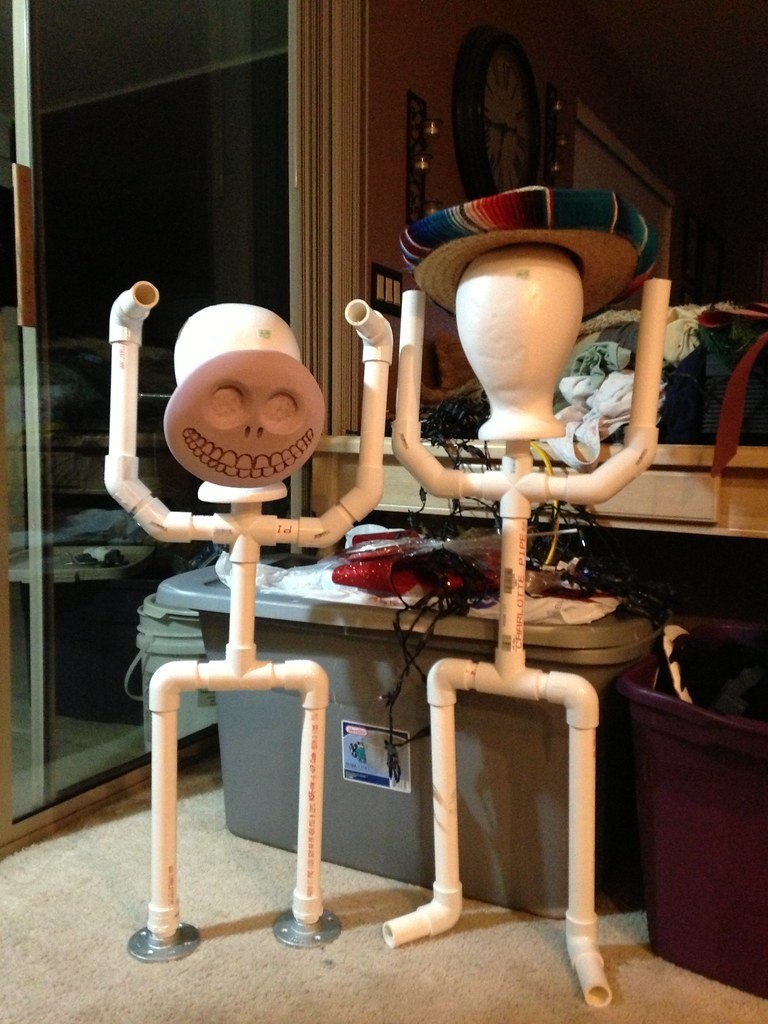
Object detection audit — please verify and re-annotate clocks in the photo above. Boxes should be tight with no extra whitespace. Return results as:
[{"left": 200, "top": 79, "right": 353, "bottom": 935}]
[{"left": 453, "top": 18, "right": 541, "bottom": 201}]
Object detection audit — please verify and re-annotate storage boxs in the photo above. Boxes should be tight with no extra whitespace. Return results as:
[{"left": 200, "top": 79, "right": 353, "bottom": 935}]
[
  {"left": 613, "top": 617, "right": 768, "bottom": 1000},
  {"left": 159, "top": 553, "right": 679, "bottom": 919}
]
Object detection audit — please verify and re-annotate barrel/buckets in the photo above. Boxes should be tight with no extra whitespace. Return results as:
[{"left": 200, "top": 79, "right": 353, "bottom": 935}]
[{"left": 125, "top": 592, "right": 218, "bottom": 753}]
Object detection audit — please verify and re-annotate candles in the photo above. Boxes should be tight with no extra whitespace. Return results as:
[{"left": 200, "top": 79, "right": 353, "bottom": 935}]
[
  {"left": 416, "top": 157, "right": 429, "bottom": 170},
  {"left": 425, "top": 121, "right": 440, "bottom": 135},
  {"left": 551, "top": 161, "right": 561, "bottom": 172},
  {"left": 425, "top": 208, "right": 436, "bottom": 214},
  {"left": 559, "top": 135, "right": 567, "bottom": 145},
  {"left": 554, "top": 101, "right": 562, "bottom": 110}
]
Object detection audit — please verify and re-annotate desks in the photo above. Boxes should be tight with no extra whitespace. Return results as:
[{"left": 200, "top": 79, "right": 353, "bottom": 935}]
[{"left": 310, "top": 436, "right": 768, "bottom": 561}]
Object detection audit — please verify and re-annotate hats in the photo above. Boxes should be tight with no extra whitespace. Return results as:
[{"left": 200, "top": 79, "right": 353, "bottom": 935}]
[{"left": 400, "top": 184, "right": 658, "bottom": 324}]
